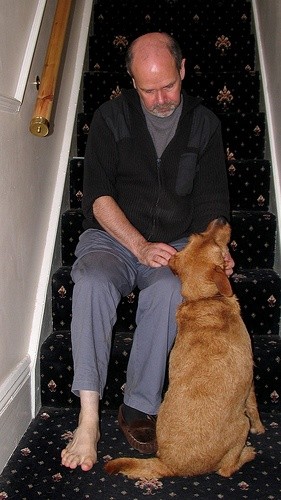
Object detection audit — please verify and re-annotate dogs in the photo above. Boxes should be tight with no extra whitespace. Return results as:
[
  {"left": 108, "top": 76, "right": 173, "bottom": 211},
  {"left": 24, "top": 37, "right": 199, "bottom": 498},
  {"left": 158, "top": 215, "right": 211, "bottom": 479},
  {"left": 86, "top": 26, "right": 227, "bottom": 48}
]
[{"left": 102, "top": 215, "right": 266, "bottom": 481}]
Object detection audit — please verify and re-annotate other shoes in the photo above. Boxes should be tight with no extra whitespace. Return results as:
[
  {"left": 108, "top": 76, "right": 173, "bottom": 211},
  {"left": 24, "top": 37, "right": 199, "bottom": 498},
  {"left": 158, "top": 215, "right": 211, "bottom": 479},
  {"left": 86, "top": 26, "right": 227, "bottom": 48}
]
[{"left": 117, "top": 403, "right": 160, "bottom": 454}]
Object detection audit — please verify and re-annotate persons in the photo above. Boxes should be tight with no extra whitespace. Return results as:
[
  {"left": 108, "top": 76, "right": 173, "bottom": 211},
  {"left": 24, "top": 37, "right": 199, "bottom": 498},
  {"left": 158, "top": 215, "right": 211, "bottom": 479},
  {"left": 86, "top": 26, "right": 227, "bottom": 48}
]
[{"left": 60, "top": 31, "right": 235, "bottom": 472}]
